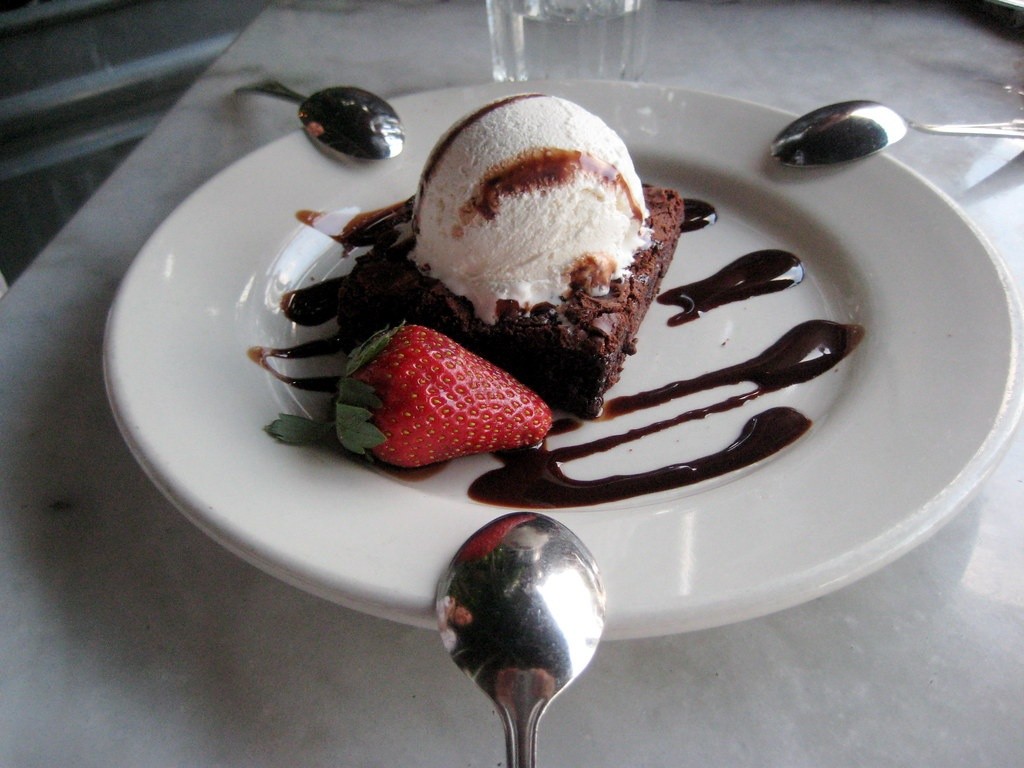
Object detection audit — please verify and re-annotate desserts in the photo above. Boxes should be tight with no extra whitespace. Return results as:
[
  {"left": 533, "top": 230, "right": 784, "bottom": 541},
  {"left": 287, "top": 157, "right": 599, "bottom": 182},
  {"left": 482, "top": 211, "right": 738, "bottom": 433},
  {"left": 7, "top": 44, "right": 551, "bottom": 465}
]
[{"left": 338, "top": 92, "right": 685, "bottom": 418}]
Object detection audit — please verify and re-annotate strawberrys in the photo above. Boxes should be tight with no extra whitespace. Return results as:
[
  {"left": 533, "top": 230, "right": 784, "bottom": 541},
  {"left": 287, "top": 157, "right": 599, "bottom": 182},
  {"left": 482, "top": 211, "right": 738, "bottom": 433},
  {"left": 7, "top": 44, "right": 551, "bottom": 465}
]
[{"left": 269, "top": 319, "right": 552, "bottom": 469}]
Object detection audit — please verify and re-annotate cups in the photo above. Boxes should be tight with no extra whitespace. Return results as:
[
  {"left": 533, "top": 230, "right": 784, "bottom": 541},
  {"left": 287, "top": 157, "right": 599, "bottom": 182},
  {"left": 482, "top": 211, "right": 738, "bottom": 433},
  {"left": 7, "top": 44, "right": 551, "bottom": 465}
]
[{"left": 486, "top": 0, "right": 659, "bottom": 87}]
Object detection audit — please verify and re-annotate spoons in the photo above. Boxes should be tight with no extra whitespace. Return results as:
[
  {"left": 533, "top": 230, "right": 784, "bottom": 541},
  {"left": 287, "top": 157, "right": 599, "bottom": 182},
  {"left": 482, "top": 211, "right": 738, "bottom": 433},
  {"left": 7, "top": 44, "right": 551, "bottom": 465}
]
[
  {"left": 433, "top": 513, "right": 605, "bottom": 768},
  {"left": 228, "top": 75, "right": 407, "bottom": 161},
  {"left": 769, "top": 96, "right": 968, "bottom": 168}
]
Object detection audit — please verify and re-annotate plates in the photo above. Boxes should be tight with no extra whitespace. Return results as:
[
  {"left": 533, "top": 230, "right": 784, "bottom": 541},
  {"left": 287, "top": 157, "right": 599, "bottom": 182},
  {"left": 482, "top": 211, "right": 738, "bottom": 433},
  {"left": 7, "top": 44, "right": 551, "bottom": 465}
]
[{"left": 105, "top": 72, "right": 1024, "bottom": 644}]
